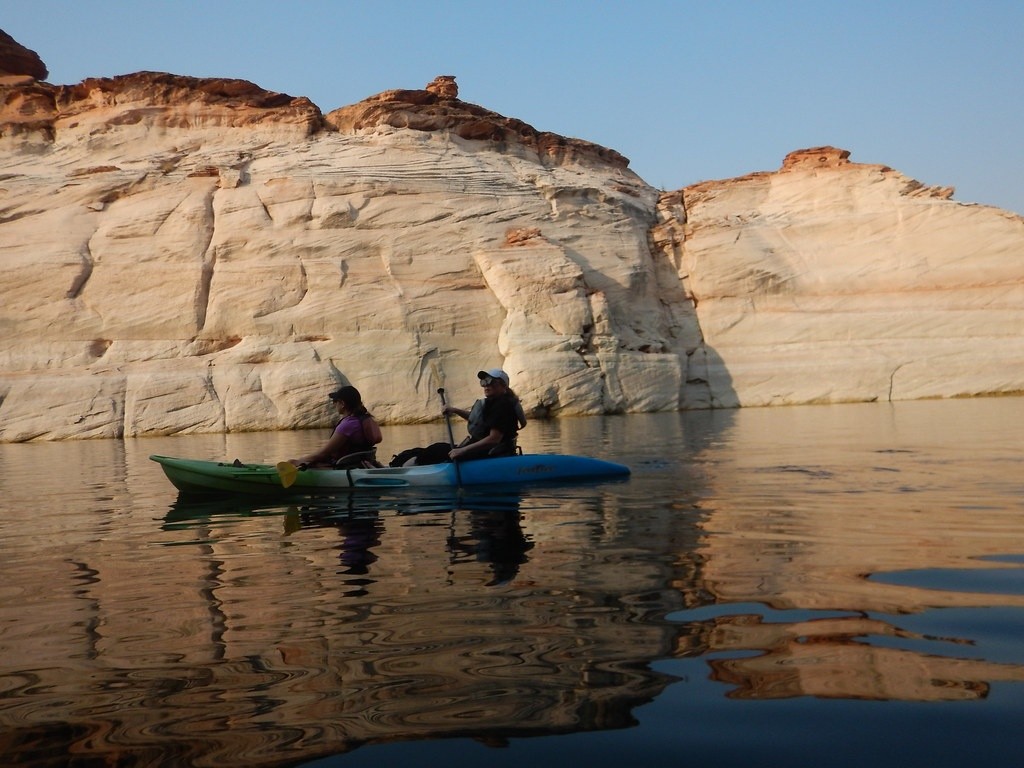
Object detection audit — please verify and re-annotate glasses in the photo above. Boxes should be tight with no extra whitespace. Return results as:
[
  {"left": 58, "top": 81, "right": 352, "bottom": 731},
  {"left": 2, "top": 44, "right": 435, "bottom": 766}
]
[{"left": 480, "top": 378, "right": 502, "bottom": 387}]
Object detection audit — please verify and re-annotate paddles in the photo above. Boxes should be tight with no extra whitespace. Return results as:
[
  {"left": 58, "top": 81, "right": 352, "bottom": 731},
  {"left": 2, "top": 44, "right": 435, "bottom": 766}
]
[
  {"left": 276, "top": 461, "right": 311, "bottom": 489},
  {"left": 437, "top": 387, "right": 462, "bottom": 490}
]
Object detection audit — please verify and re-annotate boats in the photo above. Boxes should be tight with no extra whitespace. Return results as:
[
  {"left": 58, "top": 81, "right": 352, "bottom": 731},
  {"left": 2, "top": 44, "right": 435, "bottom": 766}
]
[{"left": 149, "top": 451, "right": 633, "bottom": 498}]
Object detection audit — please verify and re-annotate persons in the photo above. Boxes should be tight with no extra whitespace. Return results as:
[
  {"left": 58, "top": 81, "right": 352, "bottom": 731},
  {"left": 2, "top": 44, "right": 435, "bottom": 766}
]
[
  {"left": 233, "top": 386, "right": 373, "bottom": 470},
  {"left": 362, "top": 368, "right": 518, "bottom": 468}
]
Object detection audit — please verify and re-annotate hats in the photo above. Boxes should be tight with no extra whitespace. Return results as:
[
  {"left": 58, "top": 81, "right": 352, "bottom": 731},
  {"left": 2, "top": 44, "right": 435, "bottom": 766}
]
[
  {"left": 329, "top": 386, "right": 361, "bottom": 406},
  {"left": 478, "top": 369, "right": 509, "bottom": 388}
]
